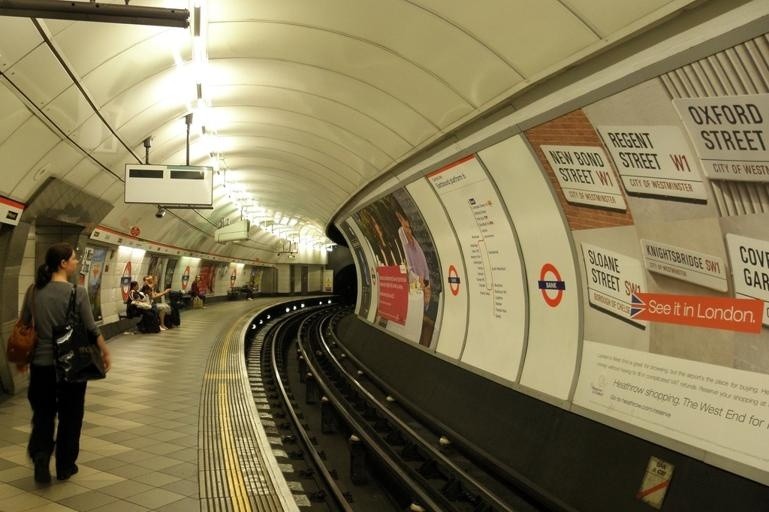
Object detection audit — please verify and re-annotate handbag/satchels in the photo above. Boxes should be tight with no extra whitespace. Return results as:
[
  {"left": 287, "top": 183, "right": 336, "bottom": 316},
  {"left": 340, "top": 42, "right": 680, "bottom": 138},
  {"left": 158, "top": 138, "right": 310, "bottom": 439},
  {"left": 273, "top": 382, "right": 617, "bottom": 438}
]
[
  {"left": 6, "top": 284, "right": 38, "bottom": 365},
  {"left": 51, "top": 284, "right": 106, "bottom": 382},
  {"left": 128, "top": 290, "right": 152, "bottom": 310},
  {"left": 192, "top": 296, "right": 204, "bottom": 308}
]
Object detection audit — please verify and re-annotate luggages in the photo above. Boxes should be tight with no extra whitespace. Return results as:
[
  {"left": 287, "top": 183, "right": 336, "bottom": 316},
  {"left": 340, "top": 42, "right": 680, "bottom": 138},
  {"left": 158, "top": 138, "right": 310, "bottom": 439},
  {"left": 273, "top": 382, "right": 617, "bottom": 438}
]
[{"left": 168, "top": 290, "right": 181, "bottom": 328}]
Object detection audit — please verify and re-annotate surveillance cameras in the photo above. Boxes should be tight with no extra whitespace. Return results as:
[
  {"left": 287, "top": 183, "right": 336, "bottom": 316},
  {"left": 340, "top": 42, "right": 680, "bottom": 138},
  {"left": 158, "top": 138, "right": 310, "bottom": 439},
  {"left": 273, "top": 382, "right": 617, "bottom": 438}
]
[{"left": 156, "top": 210, "right": 165, "bottom": 217}]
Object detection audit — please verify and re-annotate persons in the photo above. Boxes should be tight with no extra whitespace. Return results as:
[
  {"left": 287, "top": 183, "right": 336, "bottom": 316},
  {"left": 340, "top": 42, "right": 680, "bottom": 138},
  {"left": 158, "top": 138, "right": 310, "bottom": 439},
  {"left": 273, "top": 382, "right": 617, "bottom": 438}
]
[
  {"left": 7, "top": 242, "right": 111, "bottom": 482},
  {"left": 191, "top": 275, "right": 208, "bottom": 308},
  {"left": 360, "top": 209, "right": 398, "bottom": 266},
  {"left": 392, "top": 198, "right": 430, "bottom": 290},
  {"left": 126, "top": 276, "right": 172, "bottom": 334},
  {"left": 241, "top": 281, "right": 256, "bottom": 300},
  {"left": 424, "top": 289, "right": 435, "bottom": 320}
]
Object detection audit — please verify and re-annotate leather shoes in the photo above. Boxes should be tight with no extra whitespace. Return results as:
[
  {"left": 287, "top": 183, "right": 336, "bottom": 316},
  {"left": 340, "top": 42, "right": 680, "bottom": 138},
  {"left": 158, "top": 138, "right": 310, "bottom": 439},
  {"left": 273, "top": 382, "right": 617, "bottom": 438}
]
[{"left": 158, "top": 324, "right": 168, "bottom": 331}]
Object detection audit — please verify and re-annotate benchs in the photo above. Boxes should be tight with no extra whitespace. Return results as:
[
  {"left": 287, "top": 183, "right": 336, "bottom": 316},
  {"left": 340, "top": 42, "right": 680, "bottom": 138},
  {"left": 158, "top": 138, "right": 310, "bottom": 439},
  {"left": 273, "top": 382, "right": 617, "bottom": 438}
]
[
  {"left": 173, "top": 292, "right": 195, "bottom": 300},
  {"left": 229, "top": 286, "right": 255, "bottom": 298}
]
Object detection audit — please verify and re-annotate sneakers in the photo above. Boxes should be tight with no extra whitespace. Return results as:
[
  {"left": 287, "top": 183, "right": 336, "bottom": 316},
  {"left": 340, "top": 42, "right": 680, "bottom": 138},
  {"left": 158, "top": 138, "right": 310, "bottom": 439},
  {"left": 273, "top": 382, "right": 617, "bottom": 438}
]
[
  {"left": 55, "top": 461, "right": 79, "bottom": 481},
  {"left": 34, "top": 460, "right": 52, "bottom": 483},
  {"left": 248, "top": 297, "right": 253, "bottom": 300}
]
